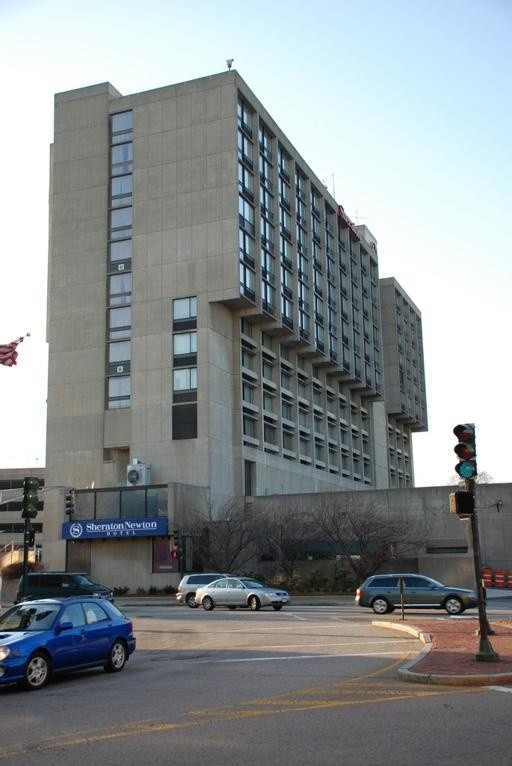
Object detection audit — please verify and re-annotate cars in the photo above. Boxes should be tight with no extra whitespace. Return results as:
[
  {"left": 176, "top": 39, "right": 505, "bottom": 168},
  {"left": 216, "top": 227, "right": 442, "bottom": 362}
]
[
  {"left": 176, "top": 573, "right": 291, "bottom": 611},
  {"left": 0, "top": 598, "right": 136, "bottom": 691},
  {"left": 355, "top": 573, "right": 479, "bottom": 614}
]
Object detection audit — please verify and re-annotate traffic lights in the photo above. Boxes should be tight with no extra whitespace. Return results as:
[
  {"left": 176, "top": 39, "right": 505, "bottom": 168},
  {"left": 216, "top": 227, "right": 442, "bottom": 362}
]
[
  {"left": 22, "top": 478, "right": 39, "bottom": 518},
  {"left": 65, "top": 494, "right": 74, "bottom": 515},
  {"left": 173, "top": 530, "right": 180, "bottom": 546},
  {"left": 454, "top": 425, "right": 477, "bottom": 479},
  {"left": 449, "top": 492, "right": 475, "bottom": 516},
  {"left": 171, "top": 550, "right": 180, "bottom": 558}
]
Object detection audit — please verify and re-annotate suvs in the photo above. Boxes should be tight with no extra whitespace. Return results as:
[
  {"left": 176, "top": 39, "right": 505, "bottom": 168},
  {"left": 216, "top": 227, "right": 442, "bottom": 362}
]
[{"left": 16, "top": 571, "right": 116, "bottom": 605}]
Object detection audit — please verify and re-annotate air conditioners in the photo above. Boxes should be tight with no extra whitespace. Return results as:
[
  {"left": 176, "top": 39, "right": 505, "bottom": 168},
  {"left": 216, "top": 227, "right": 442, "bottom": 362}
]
[
  {"left": 117, "top": 264, "right": 125, "bottom": 271},
  {"left": 126, "top": 463, "right": 151, "bottom": 486},
  {"left": 116, "top": 366, "right": 124, "bottom": 373}
]
